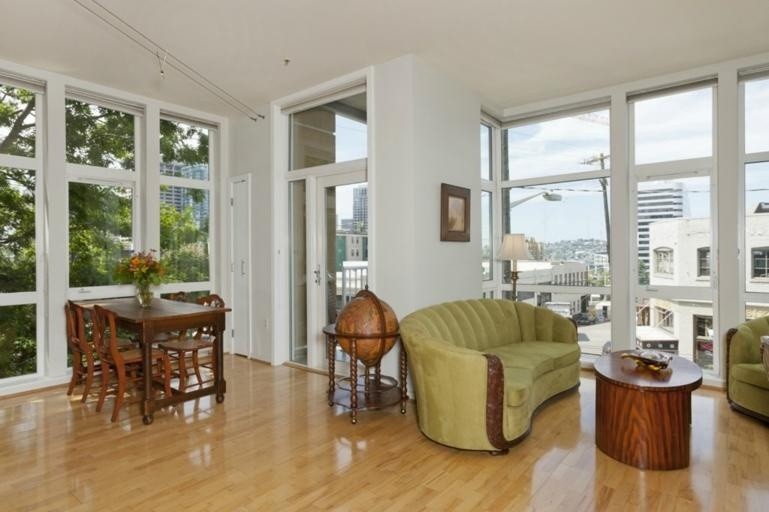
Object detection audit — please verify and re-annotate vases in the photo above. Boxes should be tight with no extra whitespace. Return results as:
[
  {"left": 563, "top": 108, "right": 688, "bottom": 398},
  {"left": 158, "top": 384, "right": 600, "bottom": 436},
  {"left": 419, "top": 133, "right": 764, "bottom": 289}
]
[{"left": 134, "top": 279, "right": 154, "bottom": 308}]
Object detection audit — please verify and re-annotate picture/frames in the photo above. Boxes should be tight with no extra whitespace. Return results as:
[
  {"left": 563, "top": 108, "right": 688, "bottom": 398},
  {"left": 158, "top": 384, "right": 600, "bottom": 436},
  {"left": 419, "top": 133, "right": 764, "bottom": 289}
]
[{"left": 441, "top": 183, "right": 470, "bottom": 242}]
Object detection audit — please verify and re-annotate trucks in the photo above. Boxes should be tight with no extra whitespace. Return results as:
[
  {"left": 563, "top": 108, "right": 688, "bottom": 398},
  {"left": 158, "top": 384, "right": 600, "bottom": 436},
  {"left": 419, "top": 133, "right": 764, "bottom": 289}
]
[{"left": 546, "top": 301, "right": 574, "bottom": 321}]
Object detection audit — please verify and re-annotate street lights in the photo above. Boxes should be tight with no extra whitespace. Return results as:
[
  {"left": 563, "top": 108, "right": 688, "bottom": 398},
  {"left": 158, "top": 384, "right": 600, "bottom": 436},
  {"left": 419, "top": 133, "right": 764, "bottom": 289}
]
[{"left": 510, "top": 191, "right": 561, "bottom": 209}]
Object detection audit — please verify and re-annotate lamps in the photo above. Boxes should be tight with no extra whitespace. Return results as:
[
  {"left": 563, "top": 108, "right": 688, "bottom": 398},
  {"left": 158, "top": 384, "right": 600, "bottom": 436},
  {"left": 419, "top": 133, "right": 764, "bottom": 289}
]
[{"left": 493, "top": 233, "right": 535, "bottom": 302}]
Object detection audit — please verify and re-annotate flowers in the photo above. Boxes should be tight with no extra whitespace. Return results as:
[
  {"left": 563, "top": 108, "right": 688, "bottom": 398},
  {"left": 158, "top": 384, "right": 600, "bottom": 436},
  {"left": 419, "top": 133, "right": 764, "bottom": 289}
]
[{"left": 115, "top": 249, "right": 170, "bottom": 303}]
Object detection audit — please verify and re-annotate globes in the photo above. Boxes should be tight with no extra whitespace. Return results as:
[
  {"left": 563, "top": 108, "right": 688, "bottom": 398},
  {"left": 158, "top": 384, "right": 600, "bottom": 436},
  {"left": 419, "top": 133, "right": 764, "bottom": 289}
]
[{"left": 322, "top": 284, "right": 407, "bottom": 424}]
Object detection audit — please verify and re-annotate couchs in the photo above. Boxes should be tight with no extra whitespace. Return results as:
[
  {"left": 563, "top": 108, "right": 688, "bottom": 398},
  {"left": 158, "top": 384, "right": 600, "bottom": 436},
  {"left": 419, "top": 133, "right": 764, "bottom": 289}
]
[{"left": 399, "top": 298, "right": 581, "bottom": 456}]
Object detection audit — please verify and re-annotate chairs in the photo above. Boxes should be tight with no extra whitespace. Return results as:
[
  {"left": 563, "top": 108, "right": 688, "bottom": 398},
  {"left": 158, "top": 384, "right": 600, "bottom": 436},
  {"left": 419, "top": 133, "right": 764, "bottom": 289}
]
[
  {"left": 725, "top": 315, "right": 769, "bottom": 425},
  {"left": 64, "top": 292, "right": 224, "bottom": 423}
]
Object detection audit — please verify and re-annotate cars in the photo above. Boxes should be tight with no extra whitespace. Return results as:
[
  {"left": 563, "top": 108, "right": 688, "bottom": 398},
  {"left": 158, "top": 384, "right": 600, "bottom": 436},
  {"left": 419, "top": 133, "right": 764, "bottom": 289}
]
[
  {"left": 574, "top": 329, "right": 611, "bottom": 357},
  {"left": 576, "top": 312, "right": 599, "bottom": 326}
]
